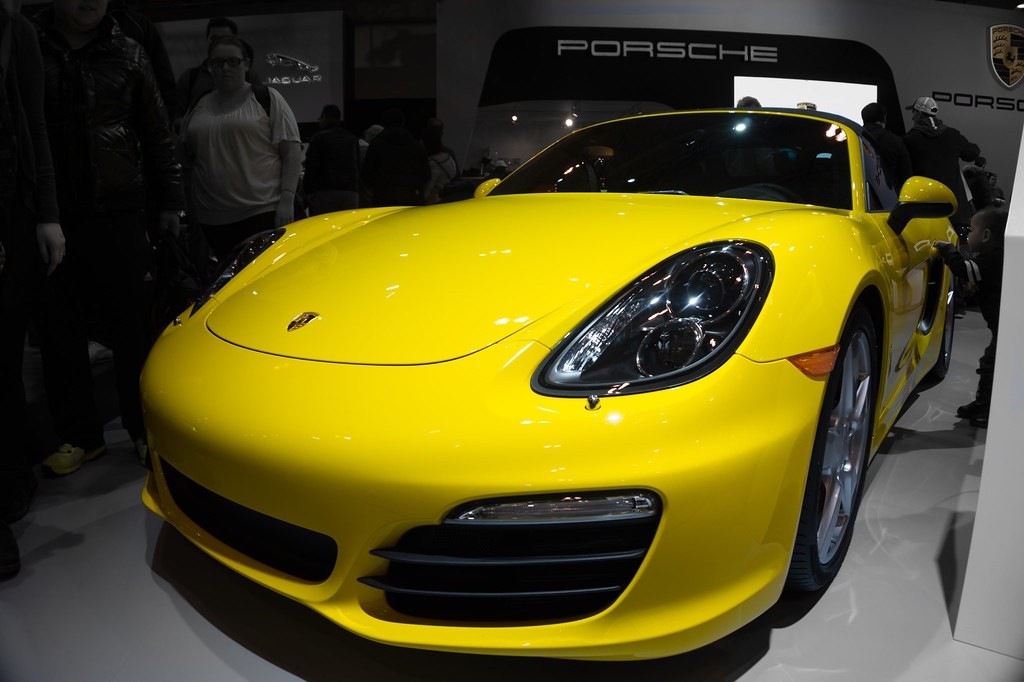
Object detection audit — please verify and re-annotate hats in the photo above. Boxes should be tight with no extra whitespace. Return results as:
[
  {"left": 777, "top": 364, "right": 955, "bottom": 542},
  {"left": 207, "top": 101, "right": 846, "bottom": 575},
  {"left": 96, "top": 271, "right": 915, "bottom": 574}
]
[
  {"left": 317, "top": 104, "right": 340, "bottom": 120},
  {"left": 428, "top": 119, "right": 443, "bottom": 130},
  {"left": 382, "top": 108, "right": 404, "bottom": 123},
  {"left": 905, "top": 96, "right": 938, "bottom": 115},
  {"left": 365, "top": 125, "right": 384, "bottom": 143}
]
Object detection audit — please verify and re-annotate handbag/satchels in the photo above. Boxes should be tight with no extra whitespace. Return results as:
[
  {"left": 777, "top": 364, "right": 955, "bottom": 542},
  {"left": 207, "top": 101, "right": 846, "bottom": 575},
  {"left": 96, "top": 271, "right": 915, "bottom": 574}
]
[
  {"left": 142, "top": 230, "right": 201, "bottom": 341},
  {"left": 445, "top": 178, "right": 474, "bottom": 202}
]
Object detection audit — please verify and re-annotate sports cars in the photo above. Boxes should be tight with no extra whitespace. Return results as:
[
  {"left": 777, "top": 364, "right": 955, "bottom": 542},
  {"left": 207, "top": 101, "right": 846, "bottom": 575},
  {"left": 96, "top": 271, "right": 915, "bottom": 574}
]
[{"left": 138, "top": 106, "right": 961, "bottom": 662}]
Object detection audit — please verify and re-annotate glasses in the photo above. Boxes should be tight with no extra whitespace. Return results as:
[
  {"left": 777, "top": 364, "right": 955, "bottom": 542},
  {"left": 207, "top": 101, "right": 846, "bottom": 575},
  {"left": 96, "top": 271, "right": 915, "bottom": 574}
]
[{"left": 208, "top": 56, "right": 245, "bottom": 66}]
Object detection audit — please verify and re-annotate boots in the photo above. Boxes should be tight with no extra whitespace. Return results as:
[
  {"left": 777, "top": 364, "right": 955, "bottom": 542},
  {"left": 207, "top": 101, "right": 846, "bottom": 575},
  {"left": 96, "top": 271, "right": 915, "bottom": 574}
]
[
  {"left": 970, "top": 411, "right": 989, "bottom": 428},
  {"left": 957, "top": 383, "right": 992, "bottom": 417}
]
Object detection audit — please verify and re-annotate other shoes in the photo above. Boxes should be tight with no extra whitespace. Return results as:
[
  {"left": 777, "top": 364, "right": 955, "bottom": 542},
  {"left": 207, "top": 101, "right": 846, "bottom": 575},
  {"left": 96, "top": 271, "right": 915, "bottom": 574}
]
[
  {"left": 954, "top": 306, "right": 966, "bottom": 317},
  {"left": 1, "top": 484, "right": 34, "bottom": 519},
  {"left": 0, "top": 520, "right": 20, "bottom": 573},
  {"left": 89, "top": 341, "right": 113, "bottom": 364}
]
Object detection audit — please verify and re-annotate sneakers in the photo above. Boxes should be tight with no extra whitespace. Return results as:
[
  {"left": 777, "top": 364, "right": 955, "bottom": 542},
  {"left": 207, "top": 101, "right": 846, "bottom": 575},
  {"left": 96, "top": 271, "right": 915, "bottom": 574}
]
[
  {"left": 133, "top": 438, "right": 151, "bottom": 464},
  {"left": 41, "top": 442, "right": 108, "bottom": 476}
]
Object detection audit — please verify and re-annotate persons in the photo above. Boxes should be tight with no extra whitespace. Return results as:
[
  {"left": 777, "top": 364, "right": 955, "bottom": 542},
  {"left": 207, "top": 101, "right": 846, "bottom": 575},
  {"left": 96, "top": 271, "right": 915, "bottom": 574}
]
[
  {"left": 738, "top": 96, "right": 761, "bottom": 109},
  {"left": 0, "top": 0, "right": 301, "bottom": 579},
  {"left": 852, "top": 103, "right": 1010, "bottom": 432},
  {"left": 304, "top": 107, "right": 460, "bottom": 216}
]
